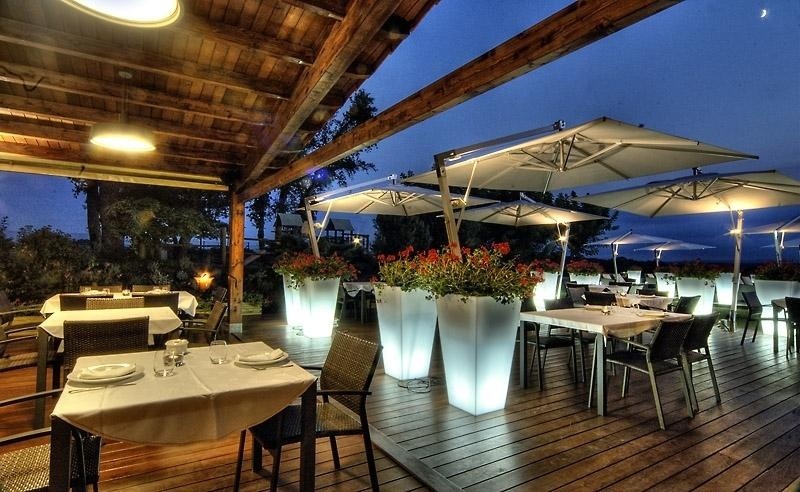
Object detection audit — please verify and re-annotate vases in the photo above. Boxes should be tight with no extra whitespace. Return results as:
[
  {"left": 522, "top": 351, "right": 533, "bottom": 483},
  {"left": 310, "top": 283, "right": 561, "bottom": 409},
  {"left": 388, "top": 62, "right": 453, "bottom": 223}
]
[
  {"left": 280, "top": 273, "right": 300, "bottom": 329},
  {"left": 432, "top": 290, "right": 524, "bottom": 417},
  {"left": 372, "top": 281, "right": 438, "bottom": 380},
  {"left": 299, "top": 275, "right": 341, "bottom": 339}
]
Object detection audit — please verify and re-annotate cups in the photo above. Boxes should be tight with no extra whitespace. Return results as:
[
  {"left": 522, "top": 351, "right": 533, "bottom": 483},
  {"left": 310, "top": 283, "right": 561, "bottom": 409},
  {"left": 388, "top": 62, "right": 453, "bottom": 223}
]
[
  {"left": 209, "top": 340, "right": 229, "bottom": 364},
  {"left": 153, "top": 347, "right": 177, "bottom": 377},
  {"left": 79, "top": 286, "right": 91, "bottom": 294},
  {"left": 122, "top": 290, "right": 130, "bottom": 296},
  {"left": 610, "top": 301, "right": 640, "bottom": 314},
  {"left": 103, "top": 288, "right": 110, "bottom": 294},
  {"left": 153, "top": 284, "right": 171, "bottom": 295},
  {"left": 165, "top": 339, "right": 189, "bottom": 355}
]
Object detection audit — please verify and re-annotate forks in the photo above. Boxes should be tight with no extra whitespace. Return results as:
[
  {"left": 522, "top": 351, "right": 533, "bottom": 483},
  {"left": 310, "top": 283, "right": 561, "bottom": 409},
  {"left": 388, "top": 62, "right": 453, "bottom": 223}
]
[{"left": 251, "top": 364, "right": 293, "bottom": 370}]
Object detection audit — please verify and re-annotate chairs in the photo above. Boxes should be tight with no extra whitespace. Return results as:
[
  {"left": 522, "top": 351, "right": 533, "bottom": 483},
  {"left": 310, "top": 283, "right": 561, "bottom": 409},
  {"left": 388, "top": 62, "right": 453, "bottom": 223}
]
[
  {"left": 232, "top": 331, "right": 384, "bottom": 492},
  {"left": 605, "top": 315, "right": 695, "bottom": 429},
  {"left": 516, "top": 276, "right": 701, "bottom": 387},
  {"left": 1, "top": 433, "right": 100, "bottom": 492},
  {"left": 625, "top": 311, "right": 721, "bottom": 412},
  {"left": 31, "top": 281, "right": 229, "bottom": 429},
  {"left": 784, "top": 296, "right": 799, "bottom": 359},
  {"left": 739, "top": 283, "right": 787, "bottom": 346}
]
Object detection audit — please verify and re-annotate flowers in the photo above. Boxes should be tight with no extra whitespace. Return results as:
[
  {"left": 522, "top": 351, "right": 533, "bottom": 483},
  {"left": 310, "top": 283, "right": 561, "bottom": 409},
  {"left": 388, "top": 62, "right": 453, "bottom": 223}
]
[
  {"left": 274, "top": 252, "right": 304, "bottom": 277},
  {"left": 368, "top": 244, "right": 433, "bottom": 292},
  {"left": 291, "top": 253, "right": 363, "bottom": 288},
  {"left": 416, "top": 241, "right": 547, "bottom": 305}
]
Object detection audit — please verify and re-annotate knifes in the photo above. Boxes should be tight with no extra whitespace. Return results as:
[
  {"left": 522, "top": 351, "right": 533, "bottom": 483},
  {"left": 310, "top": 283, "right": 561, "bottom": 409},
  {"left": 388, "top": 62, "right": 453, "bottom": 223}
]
[{"left": 68, "top": 382, "right": 136, "bottom": 395}]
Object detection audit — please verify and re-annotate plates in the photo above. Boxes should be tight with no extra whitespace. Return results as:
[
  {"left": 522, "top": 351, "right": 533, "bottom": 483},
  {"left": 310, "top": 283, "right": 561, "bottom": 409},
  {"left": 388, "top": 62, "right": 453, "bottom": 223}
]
[
  {"left": 234, "top": 353, "right": 289, "bottom": 365},
  {"left": 66, "top": 370, "right": 142, "bottom": 383}
]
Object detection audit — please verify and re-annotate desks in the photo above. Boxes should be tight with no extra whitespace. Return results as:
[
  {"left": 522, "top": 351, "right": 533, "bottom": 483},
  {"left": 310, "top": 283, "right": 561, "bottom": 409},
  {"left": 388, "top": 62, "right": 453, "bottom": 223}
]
[{"left": 341, "top": 279, "right": 375, "bottom": 324}]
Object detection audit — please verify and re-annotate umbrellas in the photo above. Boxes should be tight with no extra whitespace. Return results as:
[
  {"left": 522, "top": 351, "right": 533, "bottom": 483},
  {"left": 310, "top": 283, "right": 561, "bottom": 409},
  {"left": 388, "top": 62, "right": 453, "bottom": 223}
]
[
  {"left": 282, "top": 173, "right": 502, "bottom": 269},
  {"left": 432, "top": 188, "right": 610, "bottom": 308},
  {"left": 721, "top": 215, "right": 799, "bottom": 269},
  {"left": 568, "top": 164, "right": 800, "bottom": 325},
  {"left": 581, "top": 226, "right": 683, "bottom": 277},
  {"left": 631, "top": 236, "right": 719, "bottom": 269},
  {"left": 401, "top": 113, "right": 760, "bottom": 270}
]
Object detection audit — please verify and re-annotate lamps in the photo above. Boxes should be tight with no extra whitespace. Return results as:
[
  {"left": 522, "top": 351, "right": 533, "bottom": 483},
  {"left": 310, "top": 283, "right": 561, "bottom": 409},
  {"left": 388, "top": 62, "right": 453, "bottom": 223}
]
[
  {"left": 60, "top": 0, "right": 185, "bottom": 32},
  {"left": 84, "top": 67, "right": 159, "bottom": 158}
]
